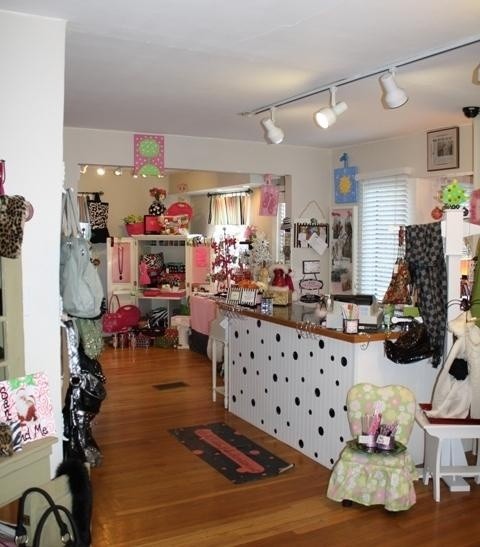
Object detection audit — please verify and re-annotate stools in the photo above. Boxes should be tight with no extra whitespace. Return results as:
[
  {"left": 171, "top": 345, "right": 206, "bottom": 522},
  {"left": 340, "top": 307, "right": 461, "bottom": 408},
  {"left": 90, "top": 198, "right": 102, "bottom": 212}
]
[{"left": 412, "top": 400, "right": 480, "bottom": 502}]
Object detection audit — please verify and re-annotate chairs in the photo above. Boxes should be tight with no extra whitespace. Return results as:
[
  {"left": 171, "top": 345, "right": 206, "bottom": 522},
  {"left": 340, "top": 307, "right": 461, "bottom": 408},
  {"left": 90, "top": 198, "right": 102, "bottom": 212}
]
[{"left": 332, "top": 383, "right": 417, "bottom": 516}]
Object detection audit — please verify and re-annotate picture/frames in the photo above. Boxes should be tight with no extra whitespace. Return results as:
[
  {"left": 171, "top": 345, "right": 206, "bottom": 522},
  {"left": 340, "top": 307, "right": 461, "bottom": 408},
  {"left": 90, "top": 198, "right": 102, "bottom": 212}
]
[{"left": 426, "top": 124, "right": 461, "bottom": 173}]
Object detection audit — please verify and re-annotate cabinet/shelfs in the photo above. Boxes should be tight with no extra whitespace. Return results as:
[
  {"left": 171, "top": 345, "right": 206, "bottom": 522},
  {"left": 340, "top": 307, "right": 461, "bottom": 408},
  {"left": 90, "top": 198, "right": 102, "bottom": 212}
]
[{"left": 107, "top": 233, "right": 202, "bottom": 329}]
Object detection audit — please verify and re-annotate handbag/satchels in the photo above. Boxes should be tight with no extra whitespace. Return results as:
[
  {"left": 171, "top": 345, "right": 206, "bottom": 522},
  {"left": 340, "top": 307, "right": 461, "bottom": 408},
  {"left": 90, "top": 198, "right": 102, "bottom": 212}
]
[
  {"left": 60, "top": 238, "right": 104, "bottom": 320},
  {"left": 65, "top": 315, "right": 105, "bottom": 384},
  {"left": 104, "top": 305, "right": 141, "bottom": 332},
  {"left": 141, "top": 252, "right": 164, "bottom": 272},
  {"left": 386, "top": 321, "right": 432, "bottom": 363},
  {"left": 88, "top": 201, "right": 108, "bottom": 230},
  {"left": 64, "top": 370, "right": 106, "bottom": 467},
  {"left": 0, "top": 195, "right": 26, "bottom": 259}
]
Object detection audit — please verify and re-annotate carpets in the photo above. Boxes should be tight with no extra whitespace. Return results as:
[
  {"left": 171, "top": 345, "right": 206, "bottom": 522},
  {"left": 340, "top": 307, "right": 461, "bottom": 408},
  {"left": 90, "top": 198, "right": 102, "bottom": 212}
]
[{"left": 164, "top": 420, "right": 296, "bottom": 485}]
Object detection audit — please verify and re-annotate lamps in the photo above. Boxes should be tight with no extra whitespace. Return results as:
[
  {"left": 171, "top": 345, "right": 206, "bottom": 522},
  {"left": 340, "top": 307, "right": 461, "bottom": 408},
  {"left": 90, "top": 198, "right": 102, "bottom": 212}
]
[{"left": 261, "top": 66, "right": 409, "bottom": 145}]
